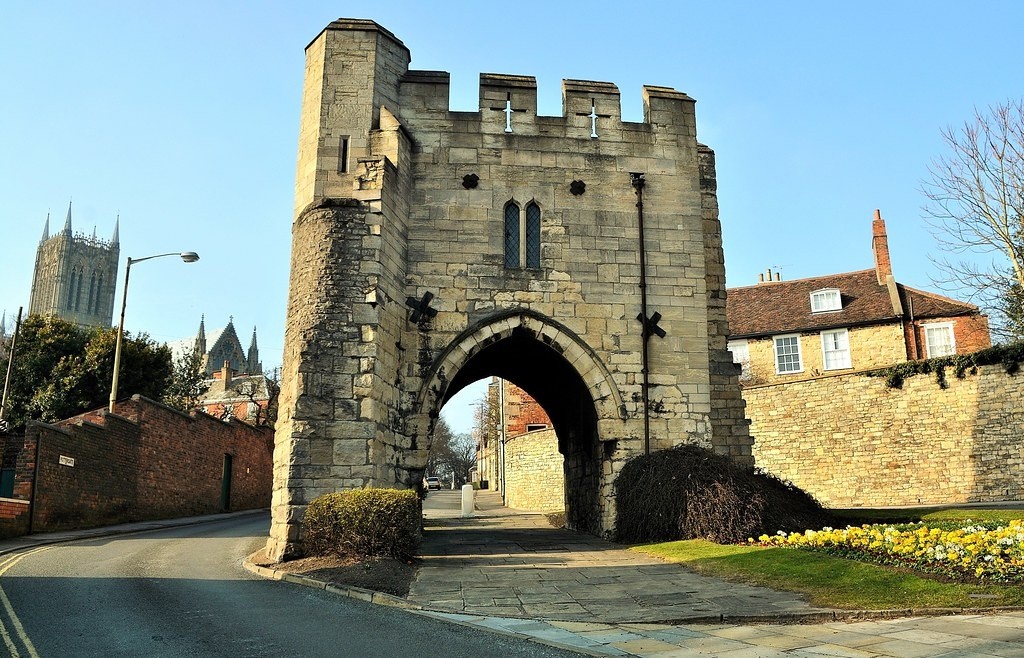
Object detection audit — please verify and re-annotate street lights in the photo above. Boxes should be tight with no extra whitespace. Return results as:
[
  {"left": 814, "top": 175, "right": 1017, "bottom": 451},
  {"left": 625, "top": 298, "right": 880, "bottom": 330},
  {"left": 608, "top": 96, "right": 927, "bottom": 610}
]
[
  {"left": 468, "top": 402, "right": 483, "bottom": 487},
  {"left": 108, "top": 251, "right": 197, "bottom": 414}
]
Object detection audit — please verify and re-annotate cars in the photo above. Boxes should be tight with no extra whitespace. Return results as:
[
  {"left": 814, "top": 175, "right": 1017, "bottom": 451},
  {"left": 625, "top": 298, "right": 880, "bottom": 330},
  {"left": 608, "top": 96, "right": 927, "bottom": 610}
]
[
  {"left": 422, "top": 476, "right": 429, "bottom": 491},
  {"left": 426, "top": 477, "right": 442, "bottom": 490}
]
